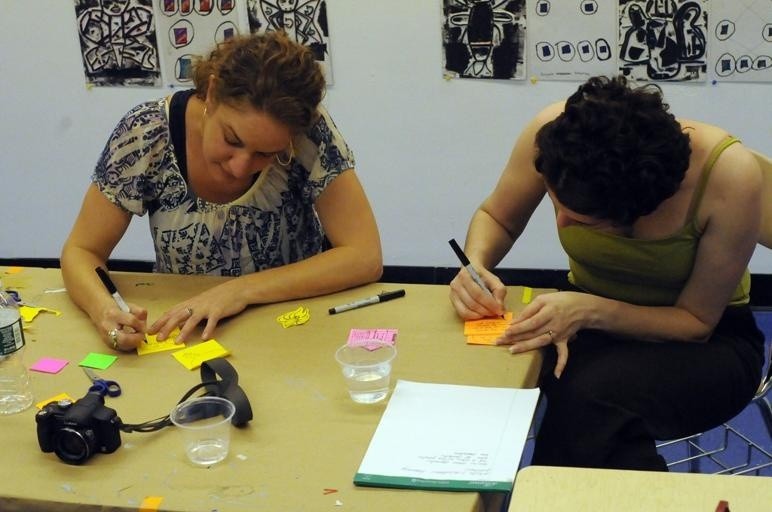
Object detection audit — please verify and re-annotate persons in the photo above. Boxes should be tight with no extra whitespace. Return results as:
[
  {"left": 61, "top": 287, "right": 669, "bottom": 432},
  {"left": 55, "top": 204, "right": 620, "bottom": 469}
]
[
  {"left": 60, "top": 31, "right": 385, "bottom": 351},
  {"left": 447, "top": 75, "right": 762, "bottom": 472}
]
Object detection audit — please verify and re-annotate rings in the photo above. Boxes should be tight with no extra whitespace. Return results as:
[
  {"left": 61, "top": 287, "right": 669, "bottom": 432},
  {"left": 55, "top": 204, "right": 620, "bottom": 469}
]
[
  {"left": 546, "top": 327, "right": 557, "bottom": 342},
  {"left": 185, "top": 306, "right": 194, "bottom": 316},
  {"left": 106, "top": 327, "right": 120, "bottom": 349}
]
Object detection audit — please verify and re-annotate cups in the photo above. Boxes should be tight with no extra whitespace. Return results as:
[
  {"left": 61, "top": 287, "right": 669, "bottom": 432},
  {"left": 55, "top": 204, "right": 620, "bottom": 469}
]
[
  {"left": 170, "top": 396, "right": 237, "bottom": 466},
  {"left": 335, "top": 339, "right": 397, "bottom": 404}
]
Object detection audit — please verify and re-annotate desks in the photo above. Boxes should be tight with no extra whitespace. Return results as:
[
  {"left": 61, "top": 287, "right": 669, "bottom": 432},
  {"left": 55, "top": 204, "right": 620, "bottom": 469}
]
[{"left": 1, "top": 266, "right": 772, "bottom": 510}]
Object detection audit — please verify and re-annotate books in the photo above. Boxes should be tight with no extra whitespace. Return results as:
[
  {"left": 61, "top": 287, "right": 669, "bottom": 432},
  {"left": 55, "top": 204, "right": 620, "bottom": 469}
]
[{"left": 353, "top": 378, "right": 541, "bottom": 494}]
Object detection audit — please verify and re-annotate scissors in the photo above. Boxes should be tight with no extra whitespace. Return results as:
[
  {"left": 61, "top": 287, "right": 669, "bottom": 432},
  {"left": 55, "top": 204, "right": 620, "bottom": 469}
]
[{"left": 83, "top": 366, "right": 121, "bottom": 397}]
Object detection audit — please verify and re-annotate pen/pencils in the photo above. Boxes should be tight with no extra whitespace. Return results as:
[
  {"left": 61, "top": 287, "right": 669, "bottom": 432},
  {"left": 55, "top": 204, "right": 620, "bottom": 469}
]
[
  {"left": 449, "top": 236, "right": 505, "bottom": 320},
  {"left": 328, "top": 290, "right": 406, "bottom": 314},
  {"left": 95, "top": 266, "right": 147, "bottom": 346}
]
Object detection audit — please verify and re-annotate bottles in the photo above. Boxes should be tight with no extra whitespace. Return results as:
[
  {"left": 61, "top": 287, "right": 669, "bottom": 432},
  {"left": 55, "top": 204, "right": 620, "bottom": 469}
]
[{"left": 0, "top": 281, "right": 33, "bottom": 415}]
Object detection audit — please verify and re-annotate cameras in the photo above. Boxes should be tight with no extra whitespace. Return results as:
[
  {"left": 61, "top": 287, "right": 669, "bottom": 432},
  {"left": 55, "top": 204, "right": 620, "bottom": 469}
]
[{"left": 35, "top": 390, "right": 122, "bottom": 465}]
